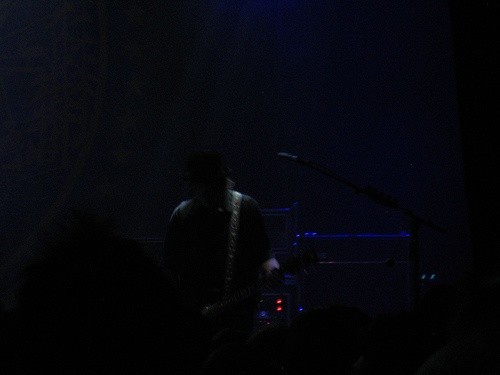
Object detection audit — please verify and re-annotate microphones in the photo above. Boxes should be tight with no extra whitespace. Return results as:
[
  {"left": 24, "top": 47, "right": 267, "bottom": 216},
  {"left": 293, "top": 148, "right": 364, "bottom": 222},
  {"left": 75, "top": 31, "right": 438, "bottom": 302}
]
[{"left": 272, "top": 151, "right": 301, "bottom": 161}]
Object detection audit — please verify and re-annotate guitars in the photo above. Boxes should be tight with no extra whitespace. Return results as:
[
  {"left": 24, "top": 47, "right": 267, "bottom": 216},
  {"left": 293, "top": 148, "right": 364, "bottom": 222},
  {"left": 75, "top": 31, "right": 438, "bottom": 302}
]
[{"left": 169, "top": 250, "right": 310, "bottom": 329}]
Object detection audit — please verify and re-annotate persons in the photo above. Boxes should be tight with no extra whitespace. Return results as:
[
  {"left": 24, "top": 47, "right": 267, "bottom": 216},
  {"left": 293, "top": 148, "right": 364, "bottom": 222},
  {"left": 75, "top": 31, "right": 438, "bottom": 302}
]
[
  {"left": 161, "top": 149, "right": 273, "bottom": 336},
  {"left": 0, "top": 274, "right": 500, "bottom": 375}
]
